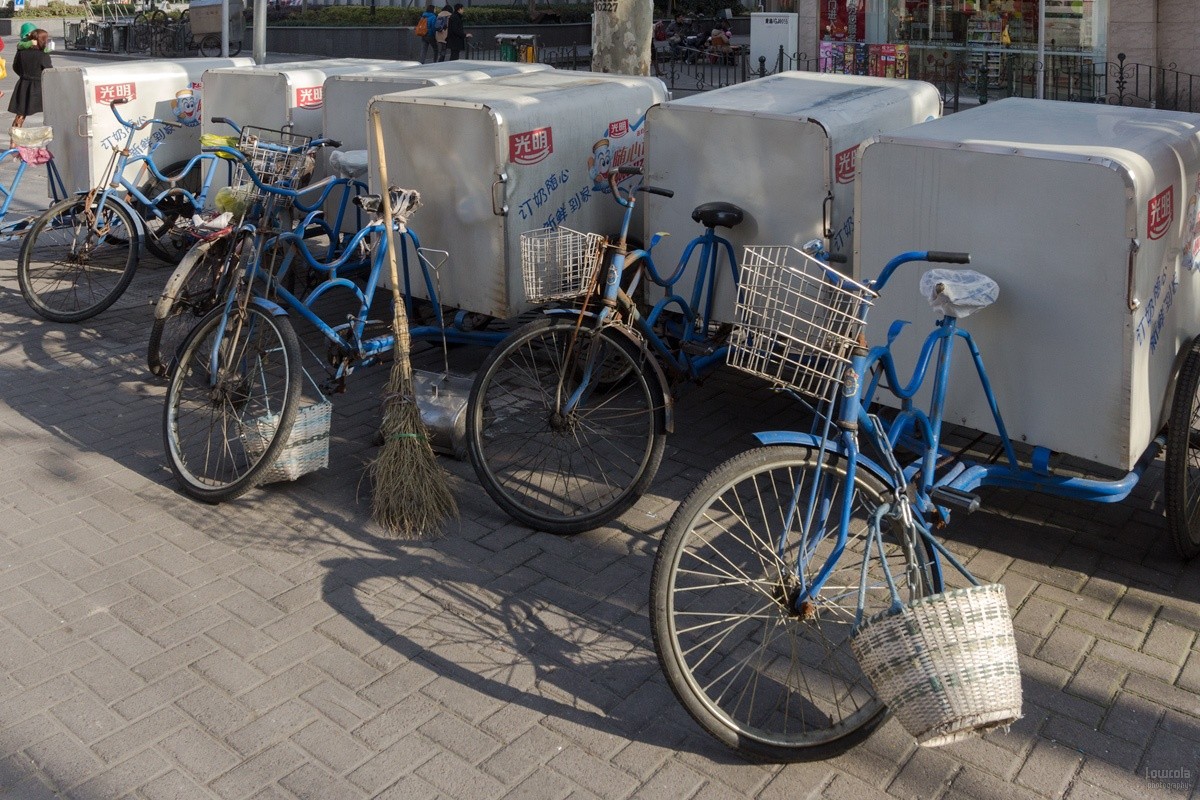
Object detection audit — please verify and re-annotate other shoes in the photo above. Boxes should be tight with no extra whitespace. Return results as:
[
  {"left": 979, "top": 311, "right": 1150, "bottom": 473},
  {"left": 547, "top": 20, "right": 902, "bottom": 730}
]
[
  {"left": 656, "top": 71, "right": 664, "bottom": 77},
  {"left": 685, "top": 60, "right": 691, "bottom": 65}
]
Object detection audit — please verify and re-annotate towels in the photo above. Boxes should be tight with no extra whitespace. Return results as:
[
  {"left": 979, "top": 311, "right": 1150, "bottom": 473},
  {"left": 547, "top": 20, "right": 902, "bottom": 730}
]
[{"left": 12, "top": 145, "right": 55, "bottom": 166}]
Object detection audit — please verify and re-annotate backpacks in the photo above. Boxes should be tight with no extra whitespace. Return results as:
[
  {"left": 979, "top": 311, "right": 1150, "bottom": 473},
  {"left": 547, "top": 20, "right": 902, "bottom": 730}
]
[
  {"left": 435, "top": 14, "right": 449, "bottom": 32},
  {"left": 415, "top": 16, "right": 429, "bottom": 36}
]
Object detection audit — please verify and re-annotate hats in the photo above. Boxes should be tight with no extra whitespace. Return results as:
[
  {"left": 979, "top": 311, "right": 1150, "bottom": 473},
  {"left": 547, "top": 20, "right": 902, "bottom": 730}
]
[{"left": 725, "top": 31, "right": 732, "bottom": 37}]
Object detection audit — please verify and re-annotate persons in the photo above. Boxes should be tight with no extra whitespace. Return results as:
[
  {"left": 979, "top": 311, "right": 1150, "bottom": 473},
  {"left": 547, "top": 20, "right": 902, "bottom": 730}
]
[
  {"left": 650, "top": 14, "right": 733, "bottom": 77},
  {"left": 437, "top": 5, "right": 454, "bottom": 62},
  {"left": 0, "top": 36, "right": 7, "bottom": 98},
  {"left": 7, "top": 23, "right": 54, "bottom": 148},
  {"left": 417, "top": 4, "right": 444, "bottom": 64},
  {"left": 445, "top": 4, "right": 473, "bottom": 62}
]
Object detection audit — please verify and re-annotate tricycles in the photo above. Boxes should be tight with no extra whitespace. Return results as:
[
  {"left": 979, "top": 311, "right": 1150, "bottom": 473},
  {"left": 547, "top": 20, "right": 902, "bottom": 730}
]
[
  {"left": 133, "top": 9, "right": 242, "bottom": 57},
  {"left": 649, "top": 238, "right": 1200, "bottom": 765},
  {"left": 163, "top": 146, "right": 649, "bottom": 504},
  {"left": 465, "top": 164, "right": 742, "bottom": 536},
  {"left": 0, "top": 99, "right": 377, "bottom": 377}
]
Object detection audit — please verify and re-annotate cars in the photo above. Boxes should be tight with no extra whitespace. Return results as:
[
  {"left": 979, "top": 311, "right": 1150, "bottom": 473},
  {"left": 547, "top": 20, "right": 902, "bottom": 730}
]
[{"left": 652, "top": 18, "right": 722, "bottom": 62}]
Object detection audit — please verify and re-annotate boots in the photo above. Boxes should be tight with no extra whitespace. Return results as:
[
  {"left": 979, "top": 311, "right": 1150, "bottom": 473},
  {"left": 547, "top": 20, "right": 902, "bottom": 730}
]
[{"left": 11, "top": 115, "right": 24, "bottom": 128}]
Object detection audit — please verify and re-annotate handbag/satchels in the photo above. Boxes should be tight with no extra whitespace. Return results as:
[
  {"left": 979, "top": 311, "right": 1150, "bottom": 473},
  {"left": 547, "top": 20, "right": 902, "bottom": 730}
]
[
  {"left": 655, "top": 22, "right": 666, "bottom": 41},
  {"left": 43, "top": 36, "right": 55, "bottom": 54}
]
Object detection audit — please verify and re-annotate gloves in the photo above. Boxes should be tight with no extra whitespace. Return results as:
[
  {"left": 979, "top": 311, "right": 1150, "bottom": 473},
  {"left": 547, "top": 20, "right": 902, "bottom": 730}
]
[{"left": 466, "top": 33, "right": 472, "bottom": 38}]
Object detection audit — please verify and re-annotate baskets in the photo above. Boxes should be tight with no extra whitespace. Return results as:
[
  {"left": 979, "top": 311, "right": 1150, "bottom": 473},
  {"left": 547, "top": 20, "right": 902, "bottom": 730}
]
[
  {"left": 240, "top": 348, "right": 332, "bottom": 486},
  {"left": 850, "top": 502, "right": 1023, "bottom": 749},
  {"left": 229, "top": 125, "right": 313, "bottom": 210},
  {"left": 519, "top": 224, "right": 606, "bottom": 304},
  {"left": 724, "top": 244, "right": 879, "bottom": 403}
]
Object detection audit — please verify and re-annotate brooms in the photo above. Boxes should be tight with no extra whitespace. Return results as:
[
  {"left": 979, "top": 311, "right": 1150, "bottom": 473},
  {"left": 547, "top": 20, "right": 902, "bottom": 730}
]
[{"left": 356, "top": 107, "right": 462, "bottom": 542}]
